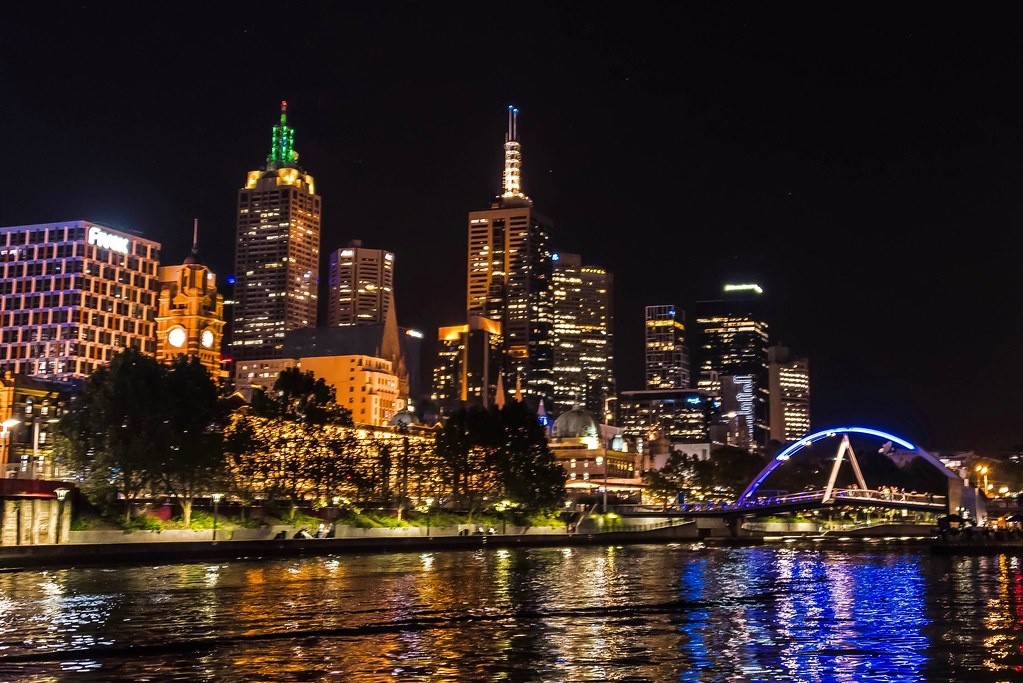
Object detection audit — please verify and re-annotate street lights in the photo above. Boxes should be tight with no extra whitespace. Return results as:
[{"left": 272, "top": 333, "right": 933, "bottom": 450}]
[
  {"left": 426, "top": 497, "right": 433, "bottom": 536},
  {"left": 53, "top": 487, "right": 70, "bottom": 544},
  {"left": 212, "top": 491, "right": 224, "bottom": 540},
  {"left": 565, "top": 500, "right": 573, "bottom": 533}
]
